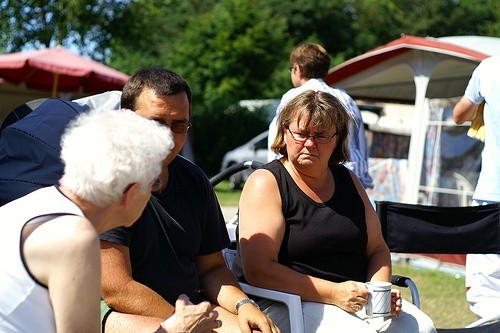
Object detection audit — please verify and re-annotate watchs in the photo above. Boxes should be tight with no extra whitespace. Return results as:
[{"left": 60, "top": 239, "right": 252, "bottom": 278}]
[{"left": 236, "top": 298, "right": 260, "bottom": 315}]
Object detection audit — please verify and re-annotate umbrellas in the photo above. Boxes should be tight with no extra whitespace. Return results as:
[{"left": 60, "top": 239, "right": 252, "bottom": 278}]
[{"left": 0, "top": 42, "right": 136, "bottom": 99}]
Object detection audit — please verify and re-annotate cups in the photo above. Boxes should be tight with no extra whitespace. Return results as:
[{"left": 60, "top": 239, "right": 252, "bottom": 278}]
[{"left": 365, "top": 281, "right": 392, "bottom": 317}]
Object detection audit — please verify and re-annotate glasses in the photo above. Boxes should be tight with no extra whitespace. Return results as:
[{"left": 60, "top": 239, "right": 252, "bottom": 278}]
[
  {"left": 151, "top": 177, "right": 162, "bottom": 192},
  {"left": 288, "top": 126, "right": 338, "bottom": 144},
  {"left": 152, "top": 119, "right": 192, "bottom": 134}
]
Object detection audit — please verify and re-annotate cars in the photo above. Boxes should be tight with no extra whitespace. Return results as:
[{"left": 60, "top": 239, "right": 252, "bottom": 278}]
[{"left": 220, "top": 129, "right": 270, "bottom": 190}]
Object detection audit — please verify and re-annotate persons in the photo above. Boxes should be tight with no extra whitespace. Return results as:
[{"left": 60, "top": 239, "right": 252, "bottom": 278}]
[
  {"left": 98, "top": 65, "right": 283, "bottom": 333},
  {"left": 452, "top": 53, "right": 499, "bottom": 319},
  {"left": 265, "top": 41, "right": 376, "bottom": 191},
  {"left": 229, "top": 90, "right": 438, "bottom": 333},
  {"left": 0, "top": 106, "right": 223, "bottom": 333}
]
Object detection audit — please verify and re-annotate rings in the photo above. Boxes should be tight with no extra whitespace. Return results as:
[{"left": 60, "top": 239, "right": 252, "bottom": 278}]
[{"left": 215, "top": 320, "right": 220, "bottom": 329}]
